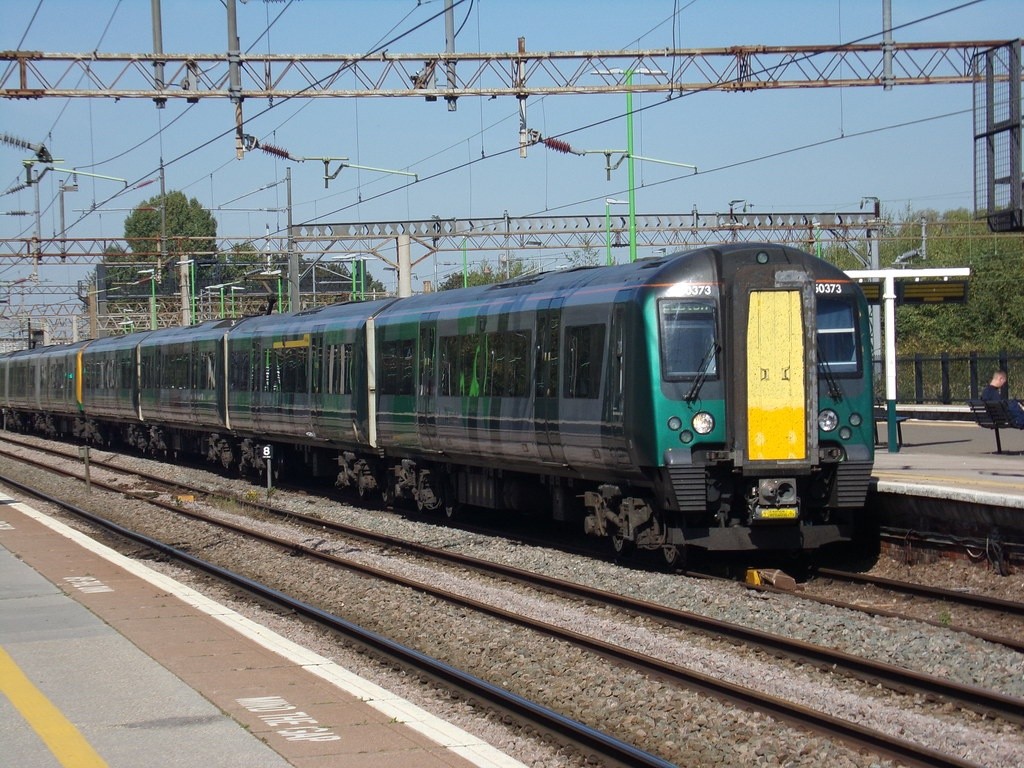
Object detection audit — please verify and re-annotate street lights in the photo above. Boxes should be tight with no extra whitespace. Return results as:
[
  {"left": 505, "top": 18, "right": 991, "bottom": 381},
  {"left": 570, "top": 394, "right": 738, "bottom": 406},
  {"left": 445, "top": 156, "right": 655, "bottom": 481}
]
[
  {"left": 590, "top": 68, "right": 669, "bottom": 262},
  {"left": 176, "top": 260, "right": 196, "bottom": 326},
  {"left": 260, "top": 270, "right": 282, "bottom": 314},
  {"left": 205, "top": 284, "right": 224, "bottom": 318},
  {"left": 137, "top": 269, "right": 157, "bottom": 330},
  {"left": 359, "top": 257, "right": 378, "bottom": 302},
  {"left": 605, "top": 197, "right": 630, "bottom": 266},
  {"left": 231, "top": 286, "right": 245, "bottom": 318},
  {"left": 331, "top": 254, "right": 357, "bottom": 302}
]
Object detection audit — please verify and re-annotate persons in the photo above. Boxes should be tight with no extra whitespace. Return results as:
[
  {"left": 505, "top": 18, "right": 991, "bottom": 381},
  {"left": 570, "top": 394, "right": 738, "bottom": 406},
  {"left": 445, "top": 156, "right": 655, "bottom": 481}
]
[{"left": 980, "top": 370, "right": 1024, "bottom": 429}]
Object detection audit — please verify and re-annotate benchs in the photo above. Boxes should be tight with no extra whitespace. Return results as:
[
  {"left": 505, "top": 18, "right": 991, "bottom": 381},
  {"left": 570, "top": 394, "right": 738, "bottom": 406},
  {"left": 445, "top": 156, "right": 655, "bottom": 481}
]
[
  {"left": 874, "top": 397, "right": 908, "bottom": 446},
  {"left": 965, "top": 398, "right": 1024, "bottom": 454}
]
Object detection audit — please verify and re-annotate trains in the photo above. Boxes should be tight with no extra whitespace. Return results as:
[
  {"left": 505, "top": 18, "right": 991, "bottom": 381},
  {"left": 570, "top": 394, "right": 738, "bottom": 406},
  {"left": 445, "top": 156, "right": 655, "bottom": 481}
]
[{"left": 0, "top": 242, "right": 879, "bottom": 578}]
